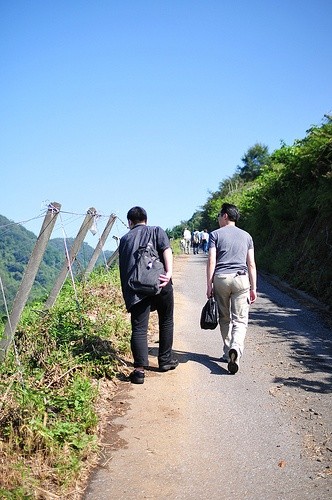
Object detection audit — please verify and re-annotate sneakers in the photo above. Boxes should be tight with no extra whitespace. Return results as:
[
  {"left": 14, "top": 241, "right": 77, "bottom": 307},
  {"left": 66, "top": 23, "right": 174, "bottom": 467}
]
[
  {"left": 222, "top": 350, "right": 231, "bottom": 363},
  {"left": 228, "top": 346, "right": 238, "bottom": 375}
]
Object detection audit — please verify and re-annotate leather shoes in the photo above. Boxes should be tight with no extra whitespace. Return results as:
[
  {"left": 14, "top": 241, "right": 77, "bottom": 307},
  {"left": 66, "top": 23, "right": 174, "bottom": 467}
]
[
  {"left": 159, "top": 359, "right": 179, "bottom": 369},
  {"left": 129, "top": 370, "right": 144, "bottom": 384}
]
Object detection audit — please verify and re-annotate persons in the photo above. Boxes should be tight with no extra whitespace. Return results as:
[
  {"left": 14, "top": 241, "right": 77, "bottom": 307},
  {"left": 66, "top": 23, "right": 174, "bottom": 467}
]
[
  {"left": 118, "top": 206, "right": 178, "bottom": 384},
  {"left": 205, "top": 203, "right": 257, "bottom": 373},
  {"left": 181, "top": 228, "right": 209, "bottom": 255}
]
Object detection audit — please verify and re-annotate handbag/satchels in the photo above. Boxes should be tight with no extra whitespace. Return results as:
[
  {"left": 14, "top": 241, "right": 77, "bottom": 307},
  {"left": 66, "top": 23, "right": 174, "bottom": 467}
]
[
  {"left": 128, "top": 242, "right": 166, "bottom": 294},
  {"left": 200, "top": 297, "right": 218, "bottom": 330}
]
